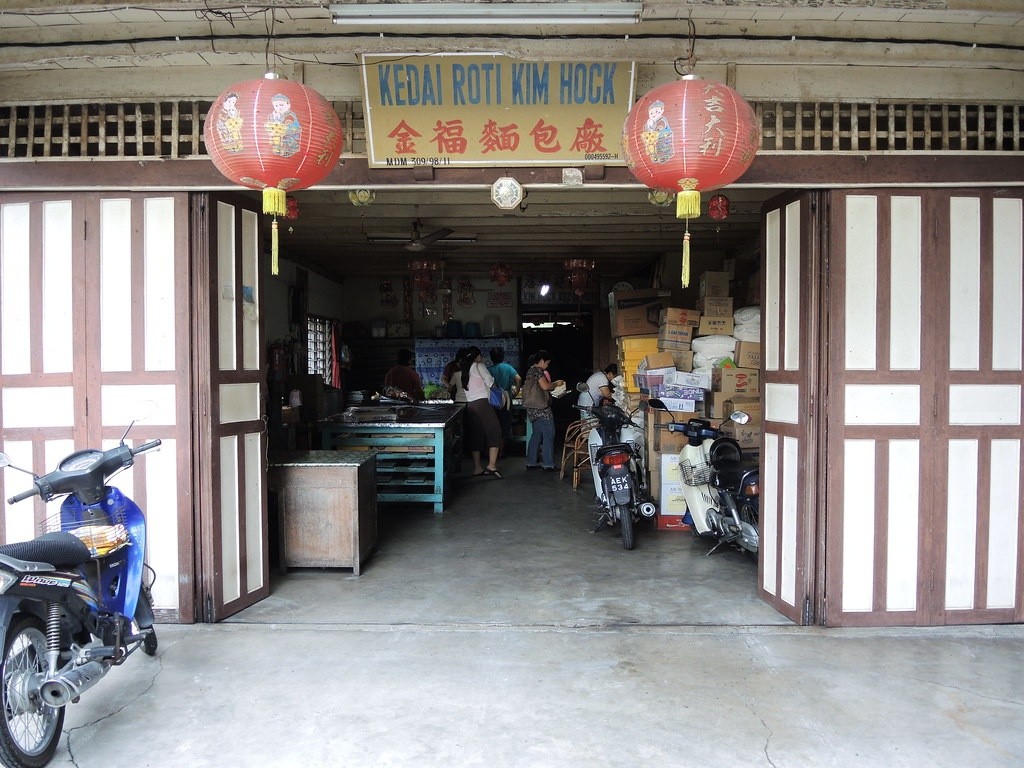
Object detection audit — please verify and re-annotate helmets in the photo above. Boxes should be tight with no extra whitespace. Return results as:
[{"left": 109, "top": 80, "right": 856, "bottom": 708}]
[{"left": 709, "top": 437, "right": 742, "bottom": 471}]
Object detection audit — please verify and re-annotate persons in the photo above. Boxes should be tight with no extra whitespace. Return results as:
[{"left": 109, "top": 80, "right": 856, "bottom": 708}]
[
  {"left": 487, "top": 347, "right": 522, "bottom": 458},
  {"left": 461, "top": 346, "right": 504, "bottom": 480},
  {"left": 577, "top": 362, "right": 617, "bottom": 467},
  {"left": 524, "top": 350, "right": 566, "bottom": 472},
  {"left": 528, "top": 354, "right": 551, "bottom": 384},
  {"left": 384, "top": 348, "right": 424, "bottom": 404},
  {"left": 443, "top": 348, "right": 468, "bottom": 418}
]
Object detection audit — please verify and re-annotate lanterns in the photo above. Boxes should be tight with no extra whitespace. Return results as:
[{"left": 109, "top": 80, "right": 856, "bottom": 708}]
[
  {"left": 620, "top": 75, "right": 760, "bottom": 289},
  {"left": 203, "top": 72, "right": 343, "bottom": 276}
]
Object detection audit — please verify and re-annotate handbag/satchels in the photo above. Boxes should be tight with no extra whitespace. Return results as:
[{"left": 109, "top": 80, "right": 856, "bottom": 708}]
[
  {"left": 489, "top": 383, "right": 510, "bottom": 410},
  {"left": 522, "top": 367, "right": 549, "bottom": 408}
]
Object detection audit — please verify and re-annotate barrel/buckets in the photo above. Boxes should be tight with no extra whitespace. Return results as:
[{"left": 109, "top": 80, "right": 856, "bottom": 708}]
[
  {"left": 436, "top": 315, "right": 501, "bottom": 339},
  {"left": 372, "top": 318, "right": 387, "bottom": 337}
]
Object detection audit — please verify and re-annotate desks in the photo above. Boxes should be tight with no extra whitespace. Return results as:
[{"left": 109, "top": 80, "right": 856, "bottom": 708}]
[
  {"left": 315, "top": 401, "right": 469, "bottom": 522},
  {"left": 270, "top": 449, "right": 380, "bottom": 579}
]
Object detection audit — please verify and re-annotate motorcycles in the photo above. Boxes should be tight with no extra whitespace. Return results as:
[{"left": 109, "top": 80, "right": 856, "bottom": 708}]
[
  {"left": 572, "top": 381, "right": 656, "bottom": 551},
  {"left": 649, "top": 398, "right": 759, "bottom": 556},
  {"left": 0, "top": 400, "right": 162, "bottom": 768}
]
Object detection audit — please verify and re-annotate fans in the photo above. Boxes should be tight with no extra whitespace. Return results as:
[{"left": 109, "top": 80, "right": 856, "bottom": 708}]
[
  {"left": 351, "top": 223, "right": 455, "bottom": 264},
  {"left": 388, "top": 260, "right": 495, "bottom": 303}
]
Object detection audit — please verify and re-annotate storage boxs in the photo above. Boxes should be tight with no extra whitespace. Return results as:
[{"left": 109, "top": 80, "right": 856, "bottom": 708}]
[
  {"left": 605, "top": 272, "right": 762, "bottom": 536},
  {"left": 370, "top": 435, "right": 445, "bottom": 454}
]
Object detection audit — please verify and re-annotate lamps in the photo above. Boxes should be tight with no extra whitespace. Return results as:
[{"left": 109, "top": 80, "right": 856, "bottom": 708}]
[{"left": 328, "top": 5, "right": 644, "bottom": 24}]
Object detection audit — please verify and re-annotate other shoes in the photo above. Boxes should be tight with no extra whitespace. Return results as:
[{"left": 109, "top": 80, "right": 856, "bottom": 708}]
[
  {"left": 484, "top": 465, "right": 504, "bottom": 479},
  {"left": 542, "top": 465, "right": 561, "bottom": 473},
  {"left": 526, "top": 464, "right": 544, "bottom": 471},
  {"left": 472, "top": 469, "right": 492, "bottom": 478}
]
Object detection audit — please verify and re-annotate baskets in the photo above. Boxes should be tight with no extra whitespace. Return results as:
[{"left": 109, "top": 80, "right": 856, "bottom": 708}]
[
  {"left": 38, "top": 505, "right": 131, "bottom": 559},
  {"left": 678, "top": 453, "right": 716, "bottom": 487},
  {"left": 633, "top": 374, "right": 663, "bottom": 389},
  {"left": 590, "top": 441, "right": 636, "bottom": 465}
]
